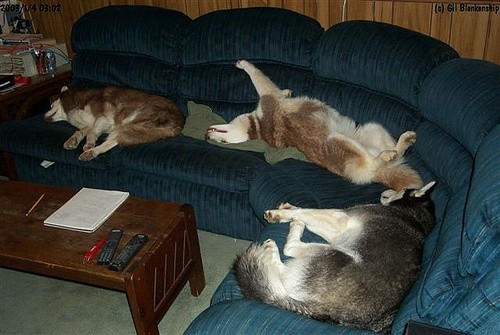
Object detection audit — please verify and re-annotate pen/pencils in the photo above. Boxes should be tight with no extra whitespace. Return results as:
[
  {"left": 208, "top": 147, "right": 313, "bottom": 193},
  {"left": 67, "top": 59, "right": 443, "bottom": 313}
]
[{"left": 26, "top": 193, "right": 45, "bottom": 216}]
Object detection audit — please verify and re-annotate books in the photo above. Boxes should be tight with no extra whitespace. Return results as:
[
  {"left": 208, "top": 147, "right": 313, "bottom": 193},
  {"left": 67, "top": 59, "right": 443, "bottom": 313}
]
[{"left": 43, "top": 187, "right": 130, "bottom": 235}]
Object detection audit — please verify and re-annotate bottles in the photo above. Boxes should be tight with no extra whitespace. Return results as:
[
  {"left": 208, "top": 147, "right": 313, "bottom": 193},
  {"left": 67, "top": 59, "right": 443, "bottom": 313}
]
[{"left": 44, "top": 50, "right": 57, "bottom": 77}]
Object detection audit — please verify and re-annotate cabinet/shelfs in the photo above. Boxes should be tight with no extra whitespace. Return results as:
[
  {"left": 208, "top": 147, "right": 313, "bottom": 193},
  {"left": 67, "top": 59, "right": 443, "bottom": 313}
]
[{"left": 0, "top": 63, "right": 73, "bottom": 181}]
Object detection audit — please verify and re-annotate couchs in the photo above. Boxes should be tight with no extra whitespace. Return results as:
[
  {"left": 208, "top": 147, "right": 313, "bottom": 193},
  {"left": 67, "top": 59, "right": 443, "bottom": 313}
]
[{"left": 0, "top": 5, "right": 500, "bottom": 335}]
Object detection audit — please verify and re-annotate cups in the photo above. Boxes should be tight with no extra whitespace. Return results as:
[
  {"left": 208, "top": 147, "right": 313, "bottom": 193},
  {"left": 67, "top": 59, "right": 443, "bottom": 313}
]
[{"left": 32, "top": 50, "right": 46, "bottom": 75}]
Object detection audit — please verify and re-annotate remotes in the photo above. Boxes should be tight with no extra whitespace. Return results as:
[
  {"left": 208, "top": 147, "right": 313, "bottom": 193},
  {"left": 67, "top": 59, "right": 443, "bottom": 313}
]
[
  {"left": 108, "top": 234, "right": 148, "bottom": 271},
  {"left": 99, "top": 228, "right": 123, "bottom": 264}
]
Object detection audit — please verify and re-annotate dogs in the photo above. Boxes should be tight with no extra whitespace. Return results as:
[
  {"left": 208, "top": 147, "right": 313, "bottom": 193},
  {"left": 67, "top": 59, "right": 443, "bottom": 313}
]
[
  {"left": 42, "top": 83, "right": 187, "bottom": 163},
  {"left": 204, "top": 58, "right": 426, "bottom": 192},
  {"left": 228, "top": 179, "right": 440, "bottom": 335}
]
